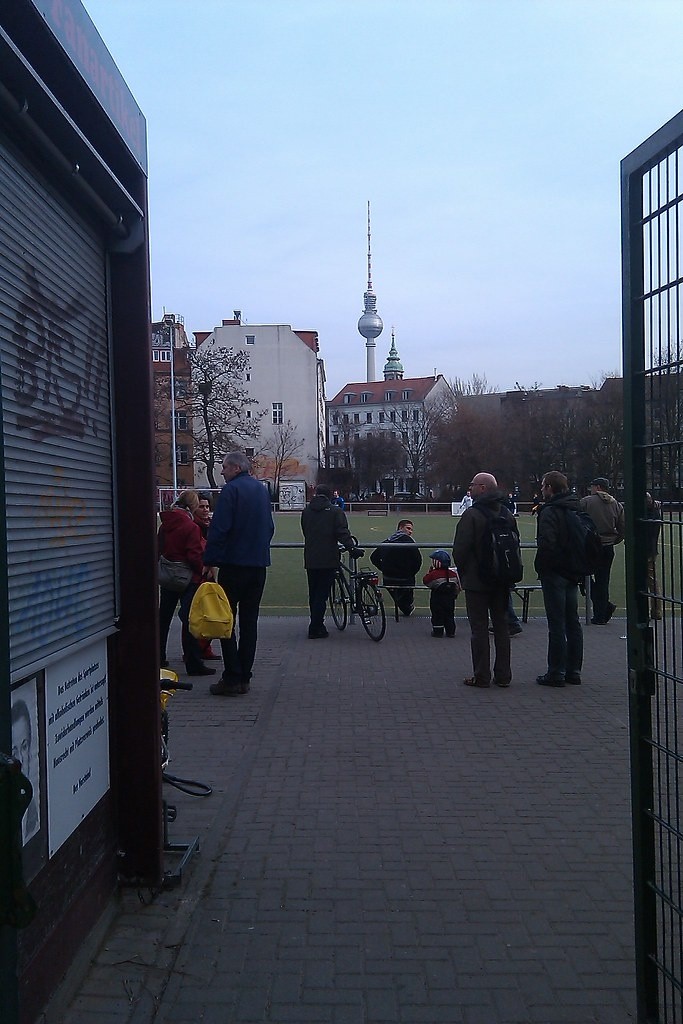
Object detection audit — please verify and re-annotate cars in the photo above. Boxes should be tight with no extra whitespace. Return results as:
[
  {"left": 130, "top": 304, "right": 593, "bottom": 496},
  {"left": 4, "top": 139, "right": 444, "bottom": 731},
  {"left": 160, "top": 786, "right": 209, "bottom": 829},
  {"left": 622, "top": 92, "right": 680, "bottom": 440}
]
[
  {"left": 359, "top": 491, "right": 386, "bottom": 503},
  {"left": 390, "top": 491, "right": 430, "bottom": 503}
]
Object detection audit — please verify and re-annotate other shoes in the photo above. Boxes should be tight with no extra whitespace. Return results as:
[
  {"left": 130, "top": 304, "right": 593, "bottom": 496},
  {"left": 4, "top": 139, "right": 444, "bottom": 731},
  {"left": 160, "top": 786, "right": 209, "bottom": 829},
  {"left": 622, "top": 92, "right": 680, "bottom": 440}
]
[
  {"left": 488, "top": 627, "right": 494, "bottom": 634},
  {"left": 591, "top": 617, "right": 608, "bottom": 625},
  {"left": 446, "top": 633, "right": 456, "bottom": 638},
  {"left": 308, "top": 630, "right": 329, "bottom": 638},
  {"left": 566, "top": 676, "right": 581, "bottom": 685},
  {"left": 187, "top": 666, "right": 216, "bottom": 675},
  {"left": 493, "top": 677, "right": 510, "bottom": 686},
  {"left": 409, "top": 598, "right": 415, "bottom": 606},
  {"left": 431, "top": 631, "right": 443, "bottom": 638},
  {"left": 407, "top": 605, "right": 415, "bottom": 616},
  {"left": 510, "top": 623, "right": 522, "bottom": 636},
  {"left": 203, "top": 653, "right": 221, "bottom": 660},
  {"left": 537, "top": 673, "right": 565, "bottom": 686},
  {"left": 464, "top": 677, "right": 490, "bottom": 688},
  {"left": 160, "top": 661, "right": 169, "bottom": 668},
  {"left": 605, "top": 603, "right": 617, "bottom": 621}
]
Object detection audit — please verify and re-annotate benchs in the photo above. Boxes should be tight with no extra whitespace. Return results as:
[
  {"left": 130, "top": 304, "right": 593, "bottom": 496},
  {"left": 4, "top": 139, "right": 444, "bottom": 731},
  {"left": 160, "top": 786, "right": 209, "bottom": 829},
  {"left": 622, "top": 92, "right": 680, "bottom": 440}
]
[
  {"left": 378, "top": 585, "right": 542, "bottom": 623},
  {"left": 368, "top": 510, "right": 387, "bottom": 516}
]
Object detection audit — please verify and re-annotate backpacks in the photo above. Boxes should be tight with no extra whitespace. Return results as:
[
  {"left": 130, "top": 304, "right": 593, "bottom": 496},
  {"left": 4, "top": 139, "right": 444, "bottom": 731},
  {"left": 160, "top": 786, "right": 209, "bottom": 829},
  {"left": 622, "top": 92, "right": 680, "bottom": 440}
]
[
  {"left": 472, "top": 503, "right": 520, "bottom": 583},
  {"left": 567, "top": 501, "right": 605, "bottom": 576},
  {"left": 188, "top": 572, "right": 234, "bottom": 640}
]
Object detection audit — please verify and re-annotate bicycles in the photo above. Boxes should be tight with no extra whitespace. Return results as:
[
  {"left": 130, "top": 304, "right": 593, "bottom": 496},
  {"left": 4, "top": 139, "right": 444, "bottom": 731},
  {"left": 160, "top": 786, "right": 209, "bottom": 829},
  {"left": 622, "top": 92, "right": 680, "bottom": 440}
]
[
  {"left": 159, "top": 667, "right": 194, "bottom": 774},
  {"left": 328, "top": 536, "right": 387, "bottom": 642}
]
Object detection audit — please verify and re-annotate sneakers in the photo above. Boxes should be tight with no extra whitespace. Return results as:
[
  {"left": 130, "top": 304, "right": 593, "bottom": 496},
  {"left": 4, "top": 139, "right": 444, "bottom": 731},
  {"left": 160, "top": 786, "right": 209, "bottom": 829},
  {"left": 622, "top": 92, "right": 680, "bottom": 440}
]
[
  {"left": 210, "top": 681, "right": 238, "bottom": 696},
  {"left": 239, "top": 682, "right": 250, "bottom": 694}
]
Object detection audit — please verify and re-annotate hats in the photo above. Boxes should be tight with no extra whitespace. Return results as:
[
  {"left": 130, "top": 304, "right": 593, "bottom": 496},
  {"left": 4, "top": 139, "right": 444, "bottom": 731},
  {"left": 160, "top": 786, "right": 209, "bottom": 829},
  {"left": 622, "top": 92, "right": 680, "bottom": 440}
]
[
  {"left": 429, "top": 550, "right": 451, "bottom": 562},
  {"left": 590, "top": 478, "right": 609, "bottom": 486}
]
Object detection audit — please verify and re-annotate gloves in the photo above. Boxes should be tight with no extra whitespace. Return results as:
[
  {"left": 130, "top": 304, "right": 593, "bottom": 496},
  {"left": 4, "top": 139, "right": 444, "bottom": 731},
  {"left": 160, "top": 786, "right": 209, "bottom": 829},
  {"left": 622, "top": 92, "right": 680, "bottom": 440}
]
[{"left": 349, "top": 547, "right": 365, "bottom": 558}]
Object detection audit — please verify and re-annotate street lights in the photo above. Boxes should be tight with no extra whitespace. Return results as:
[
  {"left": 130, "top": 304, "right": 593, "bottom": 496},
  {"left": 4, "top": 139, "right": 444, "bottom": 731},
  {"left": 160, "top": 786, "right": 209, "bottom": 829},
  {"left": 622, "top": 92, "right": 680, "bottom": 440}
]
[{"left": 164, "top": 318, "right": 177, "bottom": 502}]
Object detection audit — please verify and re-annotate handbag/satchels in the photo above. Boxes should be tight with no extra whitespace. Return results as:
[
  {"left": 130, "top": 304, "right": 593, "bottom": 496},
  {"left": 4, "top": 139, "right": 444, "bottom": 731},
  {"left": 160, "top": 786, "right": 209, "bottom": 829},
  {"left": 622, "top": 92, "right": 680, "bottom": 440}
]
[{"left": 158, "top": 555, "right": 192, "bottom": 592}]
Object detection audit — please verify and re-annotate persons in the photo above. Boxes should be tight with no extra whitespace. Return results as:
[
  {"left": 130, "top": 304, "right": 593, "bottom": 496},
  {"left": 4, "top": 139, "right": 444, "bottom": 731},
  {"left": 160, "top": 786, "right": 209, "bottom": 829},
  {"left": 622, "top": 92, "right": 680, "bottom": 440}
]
[
  {"left": 506, "top": 493, "right": 516, "bottom": 514},
  {"left": 488, "top": 589, "right": 523, "bottom": 638},
  {"left": 452, "top": 473, "right": 521, "bottom": 687},
  {"left": 157, "top": 490, "right": 222, "bottom": 675},
  {"left": 531, "top": 493, "right": 540, "bottom": 515},
  {"left": 533, "top": 470, "right": 586, "bottom": 686},
  {"left": 646, "top": 492, "right": 663, "bottom": 622},
  {"left": 301, "top": 484, "right": 365, "bottom": 639},
  {"left": 201, "top": 451, "right": 275, "bottom": 695},
  {"left": 459, "top": 491, "right": 473, "bottom": 509},
  {"left": 578, "top": 478, "right": 625, "bottom": 625},
  {"left": 369, "top": 519, "right": 422, "bottom": 615},
  {"left": 423, "top": 550, "right": 459, "bottom": 637},
  {"left": 331, "top": 489, "right": 344, "bottom": 510}
]
